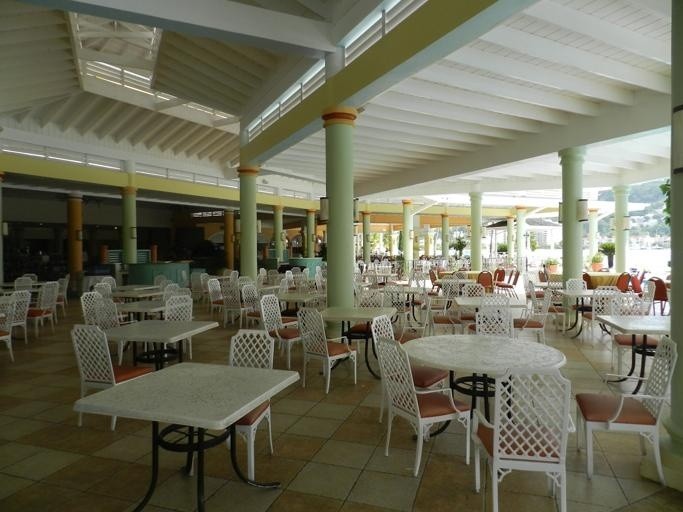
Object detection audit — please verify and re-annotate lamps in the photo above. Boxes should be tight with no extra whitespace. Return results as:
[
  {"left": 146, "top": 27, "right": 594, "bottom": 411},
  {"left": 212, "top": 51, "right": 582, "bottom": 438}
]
[
  {"left": 352, "top": 197, "right": 359, "bottom": 224},
  {"left": 557, "top": 201, "right": 562, "bottom": 222},
  {"left": 129, "top": 227, "right": 137, "bottom": 238},
  {"left": 610, "top": 216, "right": 614, "bottom": 231},
  {"left": 230, "top": 220, "right": 418, "bottom": 245},
  {"left": 622, "top": 215, "right": 632, "bottom": 232},
  {"left": 1, "top": 222, "right": 8, "bottom": 236},
  {"left": 576, "top": 198, "right": 589, "bottom": 221},
  {"left": 75, "top": 230, "right": 83, "bottom": 240},
  {"left": 318, "top": 195, "right": 328, "bottom": 222}
]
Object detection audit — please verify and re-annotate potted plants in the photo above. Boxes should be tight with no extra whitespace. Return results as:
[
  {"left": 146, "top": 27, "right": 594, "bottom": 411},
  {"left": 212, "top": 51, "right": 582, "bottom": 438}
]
[{"left": 598, "top": 241, "right": 613, "bottom": 265}]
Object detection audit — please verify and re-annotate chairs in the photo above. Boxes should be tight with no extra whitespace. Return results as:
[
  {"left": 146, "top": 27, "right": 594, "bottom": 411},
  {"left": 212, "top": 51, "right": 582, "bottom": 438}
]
[
  {"left": 375, "top": 335, "right": 472, "bottom": 477},
  {"left": 575, "top": 335, "right": 678, "bottom": 488},
  {"left": 188, "top": 328, "right": 275, "bottom": 482},
  {"left": 470, "top": 363, "right": 576, "bottom": 512},
  {"left": 0, "top": 273, "right": 70, "bottom": 363},
  {"left": 70, "top": 257, "right": 683, "bottom": 442}
]
